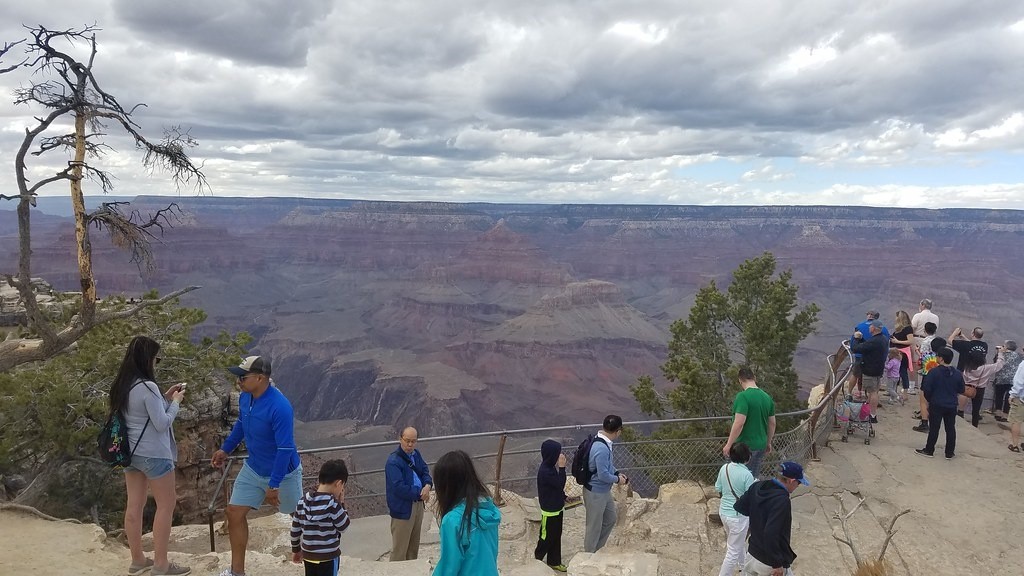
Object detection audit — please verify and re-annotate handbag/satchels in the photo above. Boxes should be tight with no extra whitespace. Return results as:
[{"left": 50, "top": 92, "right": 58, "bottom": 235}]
[
  {"left": 958, "top": 395, "right": 969, "bottom": 411},
  {"left": 837, "top": 396, "right": 870, "bottom": 421},
  {"left": 964, "top": 385, "right": 977, "bottom": 398}
]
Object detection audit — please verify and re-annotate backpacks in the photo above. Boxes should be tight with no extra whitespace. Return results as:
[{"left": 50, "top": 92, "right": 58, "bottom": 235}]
[
  {"left": 97, "top": 379, "right": 150, "bottom": 466},
  {"left": 572, "top": 434, "right": 610, "bottom": 485}
]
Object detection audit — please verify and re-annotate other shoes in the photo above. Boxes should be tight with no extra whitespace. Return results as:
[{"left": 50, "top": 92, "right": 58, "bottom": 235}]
[
  {"left": 1001, "top": 418, "right": 1007, "bottom": 422},
  {"left": 709, "top": 513, "right": 722, "bottom": 525},
  {"left": 994, "top": 415, "right": 1002, "bottom": 421},
  {"left": 845, "top": 385, "right": 964, "bottom": 423},
  {"left": 978, "top": 415, "right": 983, "bottom": 420},
  {"left": 913, "top": 410, "right": 922, "bottom": 419}
]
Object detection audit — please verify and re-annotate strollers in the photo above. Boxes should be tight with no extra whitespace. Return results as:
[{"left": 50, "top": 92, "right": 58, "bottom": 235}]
[{"left": 837, "top": 386, "right": 875, "bottom": 446}]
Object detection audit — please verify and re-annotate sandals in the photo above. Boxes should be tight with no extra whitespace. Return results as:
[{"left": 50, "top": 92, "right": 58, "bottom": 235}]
[
  {"left": 1008, "top": 443, "right": 1019, "bottom": 452},
  {"left": 1021, "top": 443, "right": 1024, "bottom": 450}
]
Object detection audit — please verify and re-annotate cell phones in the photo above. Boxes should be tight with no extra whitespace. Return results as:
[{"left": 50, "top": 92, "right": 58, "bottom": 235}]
[
  {"left": 178, "top": 383, "right": 186, "bottom": 393},
  {"left": 855, "top": 327, "right": 858, "bottom": 332}
]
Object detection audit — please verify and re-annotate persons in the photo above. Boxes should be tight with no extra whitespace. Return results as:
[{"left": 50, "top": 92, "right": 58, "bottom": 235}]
[
  {"left": 385, "top": 425, "right": 433, "bottom": 562},
  {"left": 947, "top": 327, "right": 988, "bottom": 420},
  {"left": 884, "top": 347, "right": 904, "bottom": 406},
  {"left": 962, "top": 349, "right": 1006, "bottom": 428},
  {"left": 708, "top": 368, "right": 776, "bottom": 523},
  {"left": 846, "top": 309, "right": 890, "bottom": 423},
  {"left": 107, "top": 335, "right": 191, "bottom": 576},
  {"left": 422, "top": 449, "right": 502, "bottom": 576},
  {"left": 582, "top": 415, "right": 627, "bottom": 553},
  {"left": 714, "top": 441, "right": 810, "bottom": 576},
  {"left": 913, "top": 346, "right": 965, "bottom": 459},
  {"left": 289, "top": 459, "right": 350, "bottom": 576},
  {"left": 912, "top": 336, "right": 946, "bottom": 432},
  {"left": 883, "top": 310, "right": 914, "bottom": 401},
  {"left": 993, "top": 340, "right": 1024, "bottom": 452},
  {"left": 534, "top": 439, "right": 573, "bottom": 572},
  {"left": 900, "top": 298, "right": 939, "bottom": 418},
  {"left": 210, "top": 355, "right": 304, "bottom": 576}
]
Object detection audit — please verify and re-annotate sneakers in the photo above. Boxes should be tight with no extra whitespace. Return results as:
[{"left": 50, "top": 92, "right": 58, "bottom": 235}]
[
  {"left": 128, "top": 557, "right": 154, "bottom": 575},
  {"left": 217, "top": 566, "right": 246, "bottom": 576},
  {"left": 913, "top": 424, "right": 929, "bottom": 432},
  {"left": 151, "top": 559, "right": 191, "bottom": 576},
  {"left": 945, "top": 453, "right": 955, "bottom": 459},
  {"left": 549, "top": 564, "right": 567, "bottom": 571},
  {"left": 914, "top": 448, "right": 933, "bottom": 457}
]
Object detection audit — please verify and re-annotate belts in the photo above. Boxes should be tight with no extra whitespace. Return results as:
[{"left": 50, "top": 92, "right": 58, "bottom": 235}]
[
  {"left": 913, "top": 335, "right": 925, "bottom": 339},
  {"left": 413, "top": 499, "right": 423, "bottom": 502}
]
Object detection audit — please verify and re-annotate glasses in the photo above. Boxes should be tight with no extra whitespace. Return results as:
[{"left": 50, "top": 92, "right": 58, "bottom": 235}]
[
  {"left": 239, "top": 376, "right": 252, "bottom": 382},
  {"left": 154, "top": 356, "right": 161, "bottom": 363},
  {"left": 616, "top": 427, "right": 624, "bottom": 432},
  {"left": 401, "top": 437, "right": 417, "bottom": 444}
]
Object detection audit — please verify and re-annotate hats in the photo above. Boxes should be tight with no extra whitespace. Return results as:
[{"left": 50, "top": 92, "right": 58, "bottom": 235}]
[
  {"left": 778, "top": 462, "right": 810, "bottom": 486},
  {"left": 227, "top": 356, "right": 272, "bottom": 376},
  {"left": 866, "top": 319, "right": 883, "bottom": 329}
]
